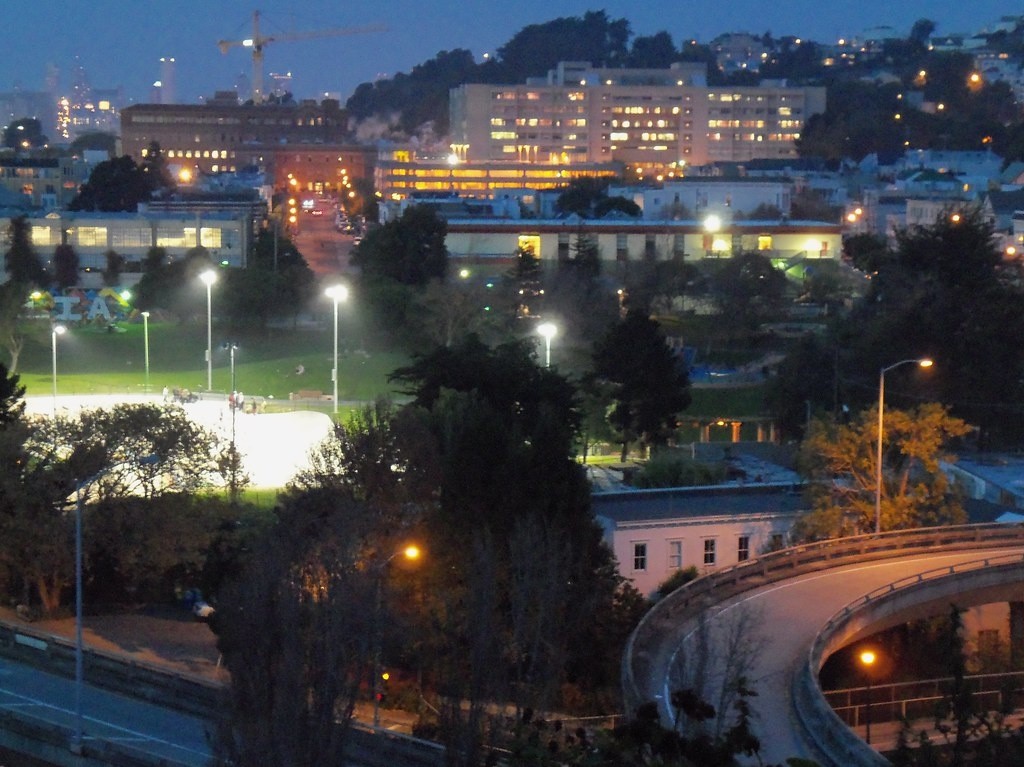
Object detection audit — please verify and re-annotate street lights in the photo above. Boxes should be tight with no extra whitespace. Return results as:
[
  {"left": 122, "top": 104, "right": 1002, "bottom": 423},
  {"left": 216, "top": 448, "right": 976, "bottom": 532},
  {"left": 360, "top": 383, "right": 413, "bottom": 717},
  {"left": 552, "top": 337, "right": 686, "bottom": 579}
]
[
  {"left": 374, "top": 543, "right": 419, "bottom": 644},
  {"left": 199, "top": 269, "right": 218, "bottom": 392},
  {"left": 325, "top": 285, "right": 350, "bottom": 412},
  {"left": 223, "top": 341, "right": 239, "bottom": 490},
  {"left": 51, "top": 325, "right": 65, "bottom": 397},
  {"left": 539, "top": 323, "right": 556, "bottom": 369},
  {"left": 142, "top": 311, "right": 151, "bottom": 390},
  {"left": 373, "top": 650, "right": 390, "bottom": 726},
  {"left": 861, "top": 650, "right": 877, "bottom": 745},
  {"left": 71, "top": 453, "right": 162, "bottom": 761},
  {"left": 876, "top": 358, "right": 932, "bottom": 536}
]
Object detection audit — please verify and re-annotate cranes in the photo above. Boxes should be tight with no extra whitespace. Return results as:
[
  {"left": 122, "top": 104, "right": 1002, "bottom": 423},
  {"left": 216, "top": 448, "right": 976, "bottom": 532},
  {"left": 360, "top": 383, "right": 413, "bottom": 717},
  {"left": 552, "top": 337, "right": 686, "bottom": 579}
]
[{"left": 219, "top": 10, "right": 387, "bottom": 104}]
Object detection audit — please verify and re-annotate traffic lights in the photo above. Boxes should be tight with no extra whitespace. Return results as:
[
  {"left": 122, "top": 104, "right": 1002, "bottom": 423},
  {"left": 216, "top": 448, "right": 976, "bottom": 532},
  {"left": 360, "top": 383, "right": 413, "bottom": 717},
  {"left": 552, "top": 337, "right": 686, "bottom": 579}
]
[{"left": 376, "top": 691, "right": 386, "bottom": 704}]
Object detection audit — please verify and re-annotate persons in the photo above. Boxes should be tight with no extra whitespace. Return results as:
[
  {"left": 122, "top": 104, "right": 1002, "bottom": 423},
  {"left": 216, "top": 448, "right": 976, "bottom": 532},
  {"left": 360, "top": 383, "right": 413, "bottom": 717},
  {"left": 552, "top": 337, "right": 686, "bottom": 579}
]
[
  {"left": 229, "top": 390, "right": 257, "bottom": 417},
  {"left": 162, "top": 383, "right": 204, "bottom": 405}
]
